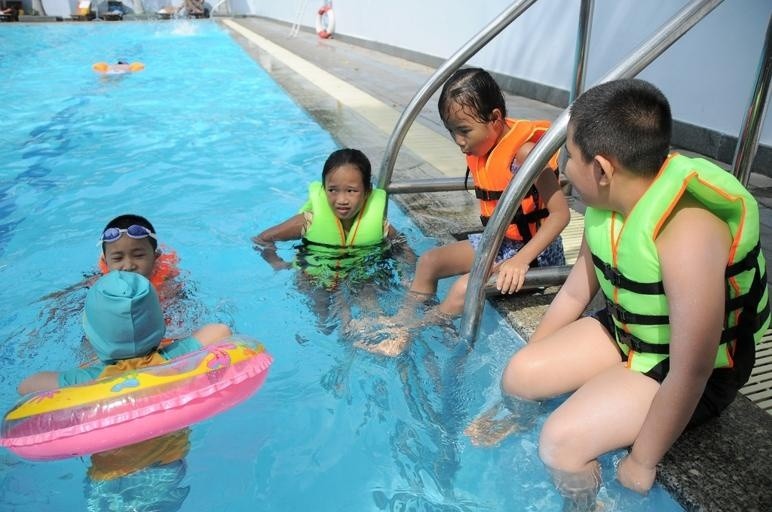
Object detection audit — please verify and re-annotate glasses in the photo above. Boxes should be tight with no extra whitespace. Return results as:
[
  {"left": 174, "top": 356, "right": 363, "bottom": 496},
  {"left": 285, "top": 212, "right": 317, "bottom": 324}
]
[{"left": 102, "top": 226, "right": 155, "bottom": 250}]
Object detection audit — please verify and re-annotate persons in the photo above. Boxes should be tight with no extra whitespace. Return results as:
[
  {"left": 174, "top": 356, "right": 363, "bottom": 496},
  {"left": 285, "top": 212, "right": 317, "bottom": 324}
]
[
  {"left": 0, "top": 0, "right": 22, "bottom": 16},
  {"left": 16, "top": 266, "right": 234, "bottom": 398},
  {"left": 249, "top": 147, "right": 420, "bottom": 336},
  {"left": 461, "top": 78, "right": 771, "bottom": 512},
  {"left": 342, "top": 67, "right": 573, "bottom": 360},
  {"left": 98, "top": 59, "right": 133, "bottom": 87},
  {"left": 185, "top": 0, "right": 204, "bottom": 13},
  {"left": 98, "top": 213, "right": 162, "bottom": 274}
]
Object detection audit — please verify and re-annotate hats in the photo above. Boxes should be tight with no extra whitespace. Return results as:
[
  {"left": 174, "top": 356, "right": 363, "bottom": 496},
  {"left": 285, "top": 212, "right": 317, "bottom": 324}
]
[{"left": 81, "top": 270, "right": 166, "bottom": 361}]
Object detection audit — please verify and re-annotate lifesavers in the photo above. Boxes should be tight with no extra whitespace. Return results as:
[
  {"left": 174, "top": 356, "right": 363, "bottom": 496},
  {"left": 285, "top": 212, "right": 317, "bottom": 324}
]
[
  {"left": 1, "top": 333, "right": 274, "bottom": 461},
  {"left": 93, "top": 62, "right": 143, "bottom": 73},
  {"left": 316, "top": 6, "right": 334, "bottom": 38}
]
